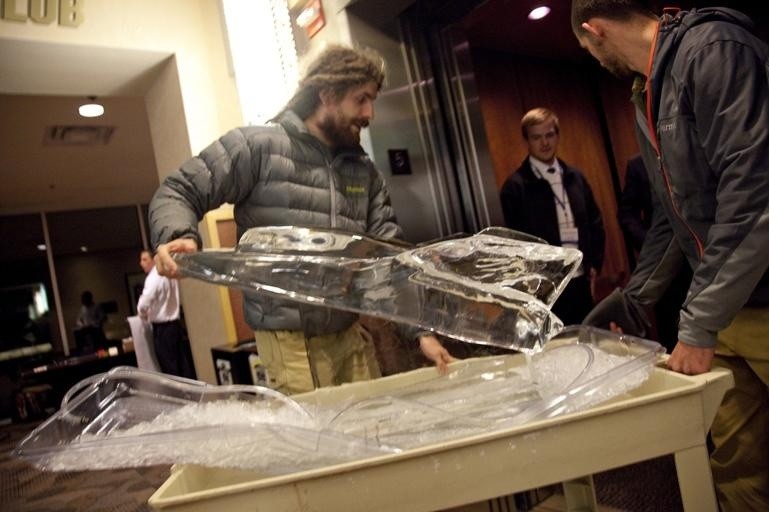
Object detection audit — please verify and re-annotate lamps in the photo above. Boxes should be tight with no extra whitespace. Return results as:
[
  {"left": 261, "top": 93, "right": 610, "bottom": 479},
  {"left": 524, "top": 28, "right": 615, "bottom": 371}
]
[{"left": 78, "top": 96, "right": 104, "bottom": 118}]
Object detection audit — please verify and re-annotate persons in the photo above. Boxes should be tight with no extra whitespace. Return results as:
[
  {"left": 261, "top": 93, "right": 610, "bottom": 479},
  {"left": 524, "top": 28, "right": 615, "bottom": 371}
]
[
  {"left": 615, "top": 153, "right": 687, "bottom": 332},
  {"left": 146, "top": 45, "right": 455, "bottom": 396},
  {"left": 76, "top": 290, "right": 97, "bottom": 327},
  {"left": 568, "top": 0, "right": 769, "bottom": 510},
  {"left": 500, "top": 107, "right": 608, "bottom": 328},
  {"left": 136, "top": 250, "right": 193, "bottom": 380}
]
